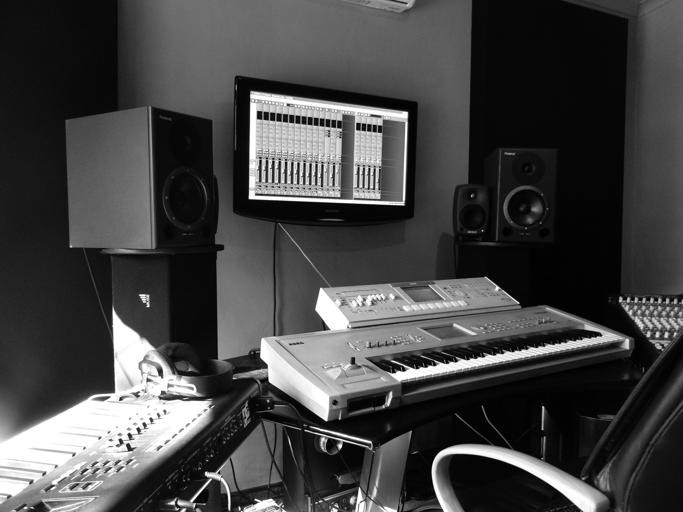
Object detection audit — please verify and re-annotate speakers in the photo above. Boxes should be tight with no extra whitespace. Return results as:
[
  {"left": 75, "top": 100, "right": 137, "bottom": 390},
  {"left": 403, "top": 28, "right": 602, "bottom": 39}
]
[
  {"left": 65, "top": 105, "right": 217, "bottom": 249},
  {"left": 453, "top": 184, "right": 489, "bottom": 239},
  {"left": 480, "top": 143, "right": 557, "bottom": 245}
]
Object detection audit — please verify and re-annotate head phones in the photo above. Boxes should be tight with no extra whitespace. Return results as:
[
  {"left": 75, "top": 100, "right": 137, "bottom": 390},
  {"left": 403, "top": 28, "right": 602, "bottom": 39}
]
[{"left": 140, "top": 340, "right": 236, "bottom": 398}]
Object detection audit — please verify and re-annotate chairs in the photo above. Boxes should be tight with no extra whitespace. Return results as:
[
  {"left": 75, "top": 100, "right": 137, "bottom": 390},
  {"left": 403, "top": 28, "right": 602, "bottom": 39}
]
[{"left": 412, "top": 329, "right": 683, "bottom": 511}]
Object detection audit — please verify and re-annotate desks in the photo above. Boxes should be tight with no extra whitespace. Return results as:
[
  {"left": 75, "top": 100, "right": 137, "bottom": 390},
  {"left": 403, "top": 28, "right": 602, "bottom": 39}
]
[{"left": 261, "top": 381, "right": 580, "bottom": 511}]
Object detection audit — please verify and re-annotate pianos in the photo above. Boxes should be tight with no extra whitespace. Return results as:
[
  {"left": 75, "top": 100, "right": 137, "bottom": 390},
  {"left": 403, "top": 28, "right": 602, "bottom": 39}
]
[
  {"left": 0, "top": 377, "right": 268, "bottom": 511},
  {"left": 260, "top": 275, "right": 635, "bottom": 421}
]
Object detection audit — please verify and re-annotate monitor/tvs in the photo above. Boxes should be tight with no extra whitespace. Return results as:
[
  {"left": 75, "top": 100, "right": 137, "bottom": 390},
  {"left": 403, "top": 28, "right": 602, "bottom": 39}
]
[{"left": 232, "top": 75, "right": 418, "bottom": 229}]
